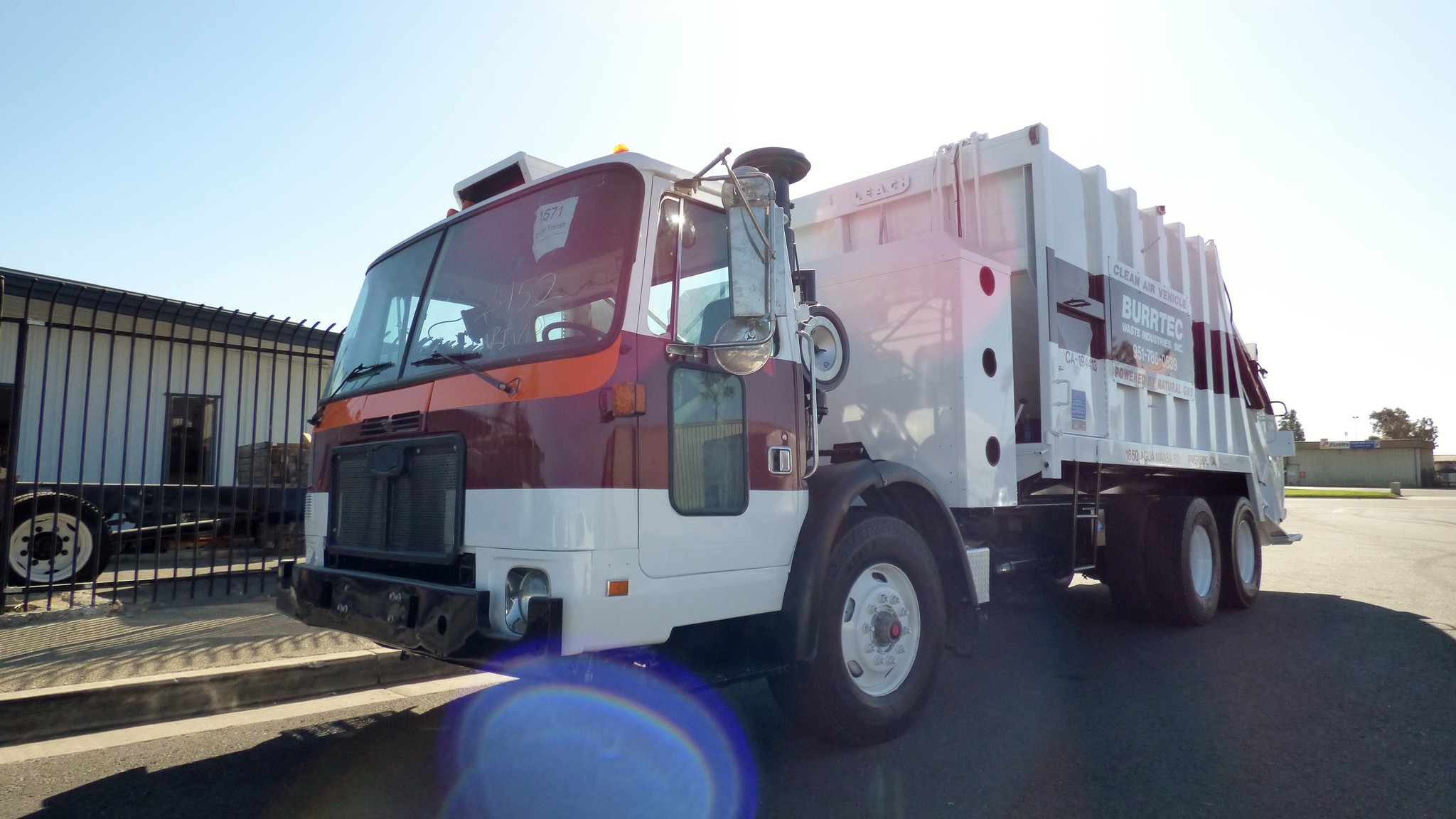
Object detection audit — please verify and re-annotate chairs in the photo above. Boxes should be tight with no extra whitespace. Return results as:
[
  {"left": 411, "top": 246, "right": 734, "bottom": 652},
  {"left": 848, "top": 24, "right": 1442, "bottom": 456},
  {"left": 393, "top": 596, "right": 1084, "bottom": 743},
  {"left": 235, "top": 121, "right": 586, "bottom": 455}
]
[{"left": 698, "top": 296, "right": 733, "bottom": 345}]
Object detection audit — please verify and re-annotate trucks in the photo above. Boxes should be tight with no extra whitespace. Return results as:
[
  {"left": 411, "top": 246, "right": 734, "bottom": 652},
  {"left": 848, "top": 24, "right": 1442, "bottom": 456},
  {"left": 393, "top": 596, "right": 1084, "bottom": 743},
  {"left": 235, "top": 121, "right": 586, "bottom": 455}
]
[{"left": 272, "top": 122, "right": 1294, "bottom": 746}]
